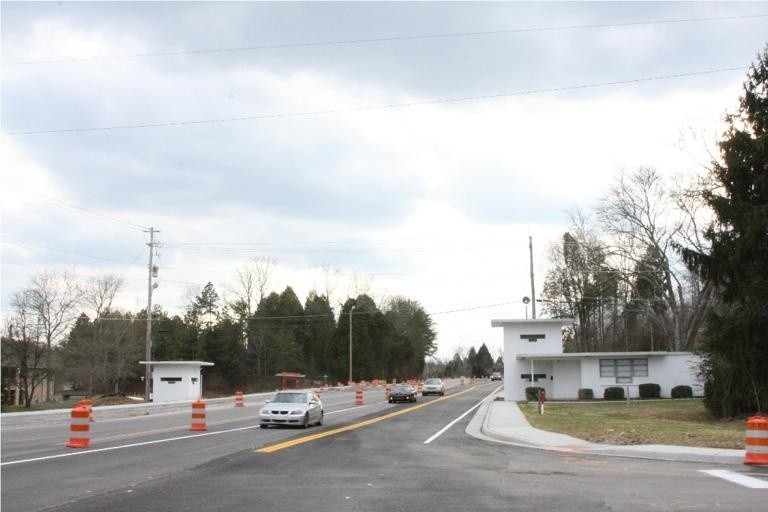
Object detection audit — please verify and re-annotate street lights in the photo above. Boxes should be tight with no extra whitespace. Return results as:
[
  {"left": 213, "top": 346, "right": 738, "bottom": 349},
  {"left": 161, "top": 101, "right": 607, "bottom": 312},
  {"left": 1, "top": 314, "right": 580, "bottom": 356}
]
[
  {"left": 146, "top": 284, "right": 158, "bottom": 400},
  {"left": 349, "top": 305, "right": 357, "bottom": 385}
]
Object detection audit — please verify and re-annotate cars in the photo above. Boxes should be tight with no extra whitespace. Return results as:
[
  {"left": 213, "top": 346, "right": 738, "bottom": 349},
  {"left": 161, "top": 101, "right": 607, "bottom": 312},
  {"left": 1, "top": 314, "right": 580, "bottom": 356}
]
[
  {"left": 389, "top": 384, "right": 418, "bottom": 403},
  {"left": 491, "top": 372, "right": 502, "bottom": 380},
  {"left": 259, "top": 389, "right": 323, "bottom": 428},
  {"left": 421, "top": 378, "right": 445, "bottom": 396}
]
